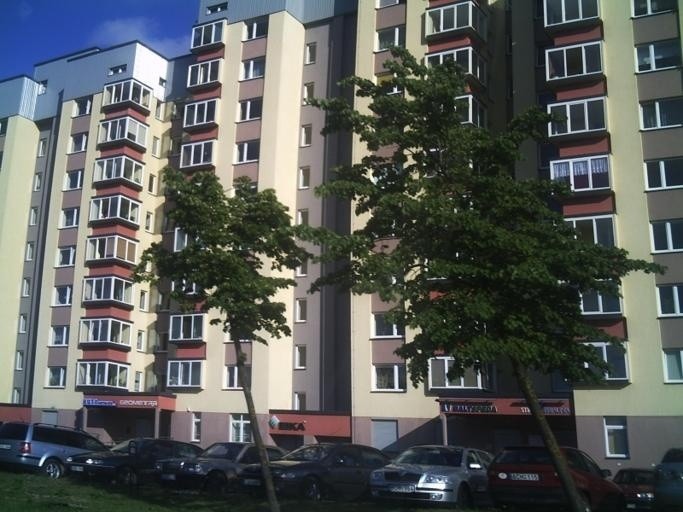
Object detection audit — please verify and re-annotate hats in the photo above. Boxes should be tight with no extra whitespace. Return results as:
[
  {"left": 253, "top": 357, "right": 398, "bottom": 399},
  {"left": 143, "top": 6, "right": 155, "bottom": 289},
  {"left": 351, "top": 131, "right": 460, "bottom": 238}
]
[
  {"left": 235, "top": 442, "right": 390, "bottom": 503},
  {"left": 63, "top": 437, "right": 204, "bottom": 491},
  {"left": 611, "top": 467, "right": 656, "bottom": 506},
  {"left": 369, "top": 445, "right": 487, "bottom": 511},
  {"left": 487, "top": 444, "right": 627, "bottom": 512},
  {"left": 153, "top": 442, "right": 288, "bottom": 500},
  {"left": 653, "top": 448, "right": 682, "bottom": 510}
]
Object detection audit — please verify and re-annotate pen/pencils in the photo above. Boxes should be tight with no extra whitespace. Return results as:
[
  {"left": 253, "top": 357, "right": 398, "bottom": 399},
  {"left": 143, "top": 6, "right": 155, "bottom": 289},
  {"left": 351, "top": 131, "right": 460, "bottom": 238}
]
[{"left": 0, "top": 419, "right": 109, "bottom": 481}]
